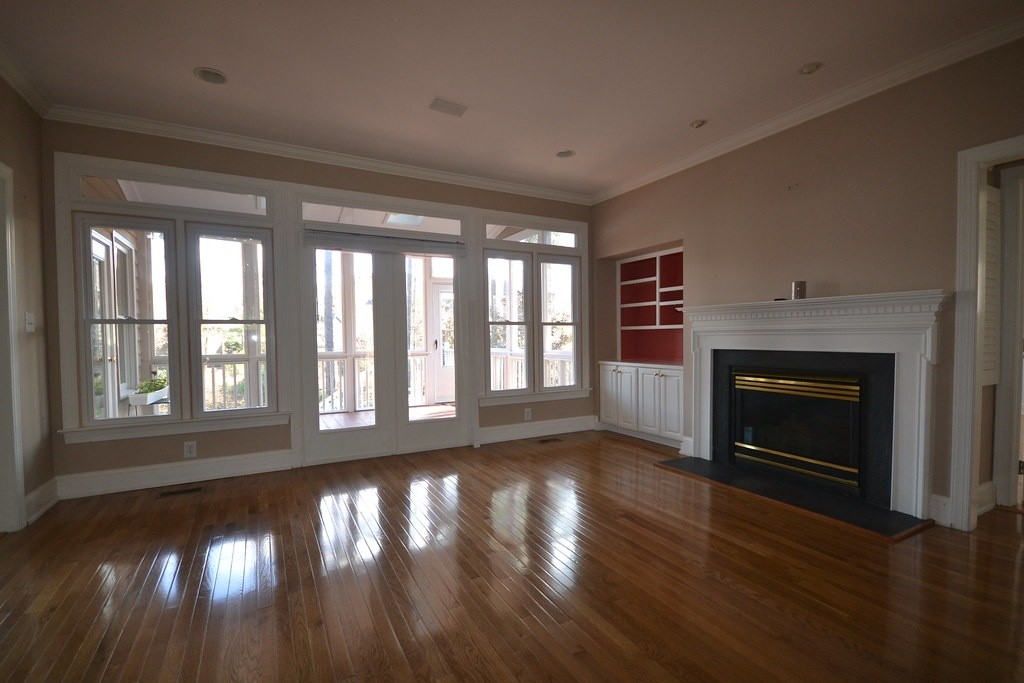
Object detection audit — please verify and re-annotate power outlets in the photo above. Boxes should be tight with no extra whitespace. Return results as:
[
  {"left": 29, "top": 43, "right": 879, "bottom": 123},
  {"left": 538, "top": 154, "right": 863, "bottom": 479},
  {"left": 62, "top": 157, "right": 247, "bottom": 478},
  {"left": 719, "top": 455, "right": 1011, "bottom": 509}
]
[
  {"left": 525, "top": 407, "right": 533, "bottom": 423},
  {"left": 183, "top": 441, "right": 197, "bottom": 460}
]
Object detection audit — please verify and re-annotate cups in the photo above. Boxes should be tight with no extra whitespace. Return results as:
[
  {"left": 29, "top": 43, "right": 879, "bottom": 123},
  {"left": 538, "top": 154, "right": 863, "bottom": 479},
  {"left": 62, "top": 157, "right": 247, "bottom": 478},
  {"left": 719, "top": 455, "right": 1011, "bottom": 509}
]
[{"left": 792, "top": 281, "right": 807, "bottom": 299}]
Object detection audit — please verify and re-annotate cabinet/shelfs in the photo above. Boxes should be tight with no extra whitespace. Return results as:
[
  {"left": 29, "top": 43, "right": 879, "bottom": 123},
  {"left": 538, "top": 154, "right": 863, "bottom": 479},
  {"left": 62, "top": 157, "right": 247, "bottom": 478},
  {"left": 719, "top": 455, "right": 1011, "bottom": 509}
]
[
  {"left": 598, "top": 364, "right": 639, "bottom": 438},
  {"left": 636, "top": 368, "right": 683, "bottom": 450}
]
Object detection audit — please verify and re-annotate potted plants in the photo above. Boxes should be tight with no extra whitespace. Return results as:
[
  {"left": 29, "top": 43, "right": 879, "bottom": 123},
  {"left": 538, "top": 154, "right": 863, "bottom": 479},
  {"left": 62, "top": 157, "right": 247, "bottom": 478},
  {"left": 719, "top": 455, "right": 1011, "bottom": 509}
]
[{"left": 129, "top": 370, "right": 170, "bottom": 405}]
